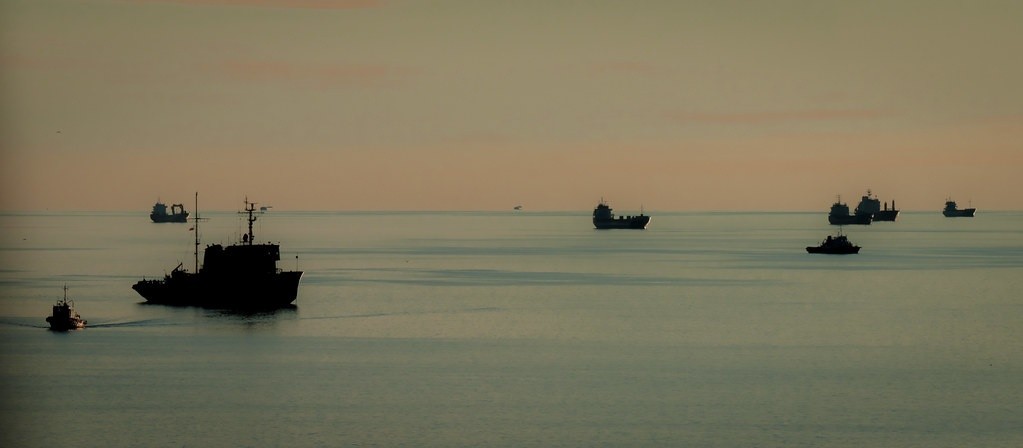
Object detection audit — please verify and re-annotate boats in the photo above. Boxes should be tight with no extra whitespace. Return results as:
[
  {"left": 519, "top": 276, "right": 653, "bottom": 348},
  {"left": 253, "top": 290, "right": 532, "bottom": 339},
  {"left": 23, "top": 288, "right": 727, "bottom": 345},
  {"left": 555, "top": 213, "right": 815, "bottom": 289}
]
[
  {"left": 804, "top": 227, "right": 863, "bottom": 255},
  {"left": 591, "top": 196, "right": 652, "bottom": 230},
  {"left": 827, "top": 194, "right": 875, "bottom": 225},
  {"left": 149, "top": 198, "right": 191, "bottom": 223},
  {"left": 45, "top": 284, "right": 88, "bottom": 332},
  {"left": 130, "top": 191, "right": 304, "bottom": 312},
  {"left": 942, "top": 195, "right": 976, "bottom": 218},
  {"left": 854, "top": 190, "right": 901, "bottom": 221}
]
